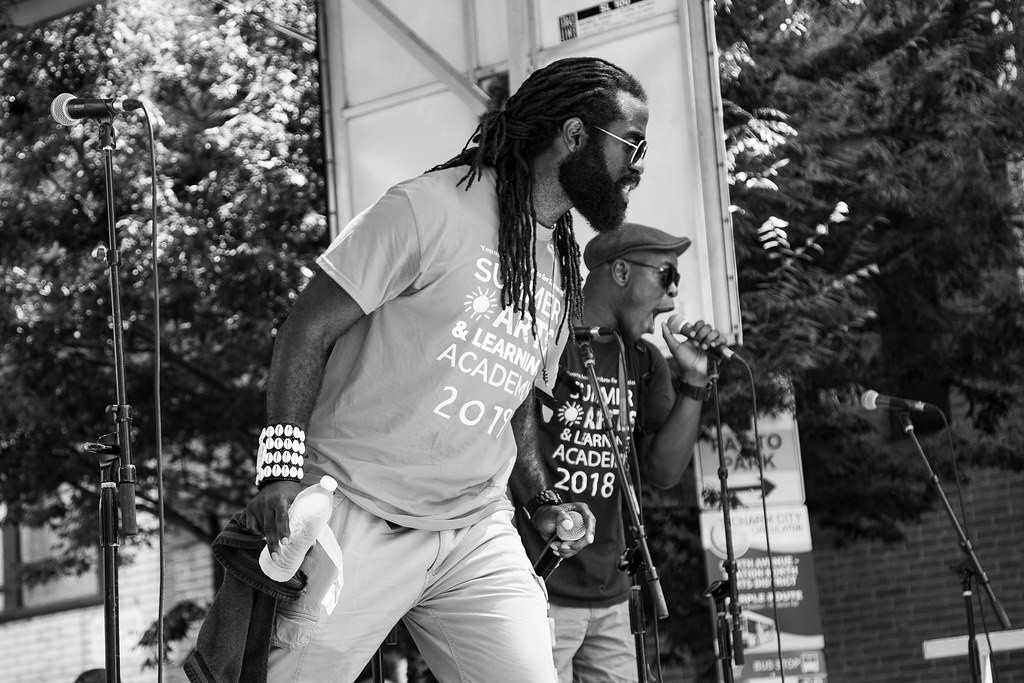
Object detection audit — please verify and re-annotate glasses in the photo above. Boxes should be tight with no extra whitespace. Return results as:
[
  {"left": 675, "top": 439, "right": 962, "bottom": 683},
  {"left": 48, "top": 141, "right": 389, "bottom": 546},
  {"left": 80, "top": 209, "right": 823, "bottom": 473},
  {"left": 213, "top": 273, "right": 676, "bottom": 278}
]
[
  {"left": 606, "top": 257, "right": 680, "bottom": 289},
  {"left": 561, "top": 119, "right": 649, "bottom": 165}
]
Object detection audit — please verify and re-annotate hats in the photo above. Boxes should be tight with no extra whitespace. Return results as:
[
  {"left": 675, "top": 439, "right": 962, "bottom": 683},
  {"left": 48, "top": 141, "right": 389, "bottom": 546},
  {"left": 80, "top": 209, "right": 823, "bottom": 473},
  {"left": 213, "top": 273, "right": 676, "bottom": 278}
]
[{"left": 583, "top": 222, "right": 692, "bottom": 270}]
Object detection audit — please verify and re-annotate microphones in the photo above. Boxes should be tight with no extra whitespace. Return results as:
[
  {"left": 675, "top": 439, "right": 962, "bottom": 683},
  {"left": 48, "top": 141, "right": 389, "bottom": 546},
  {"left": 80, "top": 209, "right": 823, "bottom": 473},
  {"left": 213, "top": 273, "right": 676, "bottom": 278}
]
[
  {"left": 861, "top": 389, "right": 937, "bottom": 413},
  {"left": 51, "top": 93, "right": 144, "bottom": 126},
  {"left": 569, "top": 326, "right": 615, "bottom": 342},
  {"left": 668, "top": 314, "right": 747, "bottom": 365},
  {"left": 533, "top": 511, "right": 587, "bottom": 582}
]
